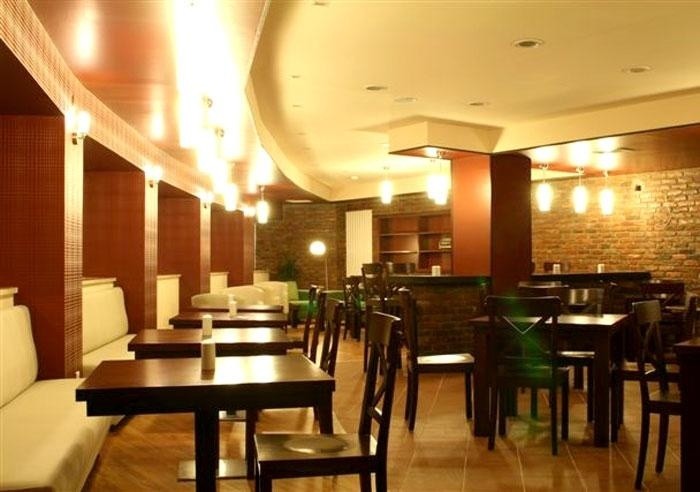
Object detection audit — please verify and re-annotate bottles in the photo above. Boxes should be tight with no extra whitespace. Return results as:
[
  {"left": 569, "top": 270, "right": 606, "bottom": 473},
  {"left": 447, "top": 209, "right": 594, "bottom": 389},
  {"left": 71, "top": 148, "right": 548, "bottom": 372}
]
[
  {"left": 228, "top": 301, "right": 237, "bottom": 317},
  {"left": 201, "top": 340, "right": 215, "bottom": 375},
  {"left": 226, "top": 294, "right": 235, "bottom": 307},
  {"left": 202, "top": 315, "right": 213, "bottom": 339}
]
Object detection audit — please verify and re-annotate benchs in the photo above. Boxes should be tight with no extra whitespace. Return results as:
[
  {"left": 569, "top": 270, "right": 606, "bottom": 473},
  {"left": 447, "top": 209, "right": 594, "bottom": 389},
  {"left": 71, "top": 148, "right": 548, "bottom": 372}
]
[
  {"left": 81, "top": 275, "right": 140, "bottom": 430},
  {"left": 157, "top": 274, "right": 182, "bottom": 331},
  {"left": 210, "top": 271, "right": 229, "bottom": 293},
  {"left": 0, "top": 285, "right": 112, "bottom": 492}
]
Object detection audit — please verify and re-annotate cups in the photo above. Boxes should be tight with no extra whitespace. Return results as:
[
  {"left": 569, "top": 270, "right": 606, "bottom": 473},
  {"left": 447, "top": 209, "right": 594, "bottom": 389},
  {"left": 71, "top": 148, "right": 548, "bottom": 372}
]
[
  {"left": 431, "top": 266, "right": 440, "bottom": 276},
  {"left": 552, "top": 263, "right": 561, "bottom": 274},
  {"left": 597, "top": 263, "right": 605, "bottom": 273}
]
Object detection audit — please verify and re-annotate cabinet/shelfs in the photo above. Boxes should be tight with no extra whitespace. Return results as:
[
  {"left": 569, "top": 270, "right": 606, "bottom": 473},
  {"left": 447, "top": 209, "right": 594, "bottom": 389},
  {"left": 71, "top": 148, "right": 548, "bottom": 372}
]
[{"left": 372, "top": 210, "right": 453, "bottom": 275}]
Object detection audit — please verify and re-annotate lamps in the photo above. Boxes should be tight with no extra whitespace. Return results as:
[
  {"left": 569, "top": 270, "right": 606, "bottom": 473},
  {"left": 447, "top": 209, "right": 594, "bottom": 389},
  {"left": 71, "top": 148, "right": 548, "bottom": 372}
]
[
  {"left": 379, "top": 137, "right": 621, "bottom": 217},
  {"left": 68, "top": 110, "right": 92, "bottom": 145},
  {"left": 146, "top": 165, "right": 164, "bottom": 188},
  {"left": 203, "top": 192, "right": 214, "bottom": 208},
  {"left": 255, "top": 185, "right": 270, "bottom": 226}
]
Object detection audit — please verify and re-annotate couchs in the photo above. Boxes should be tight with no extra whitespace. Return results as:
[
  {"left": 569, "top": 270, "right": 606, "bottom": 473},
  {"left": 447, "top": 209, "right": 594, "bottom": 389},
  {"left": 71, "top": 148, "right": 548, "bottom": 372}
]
[{"left": 190, "top": 281, "right": 289, "bottom": 314}]
